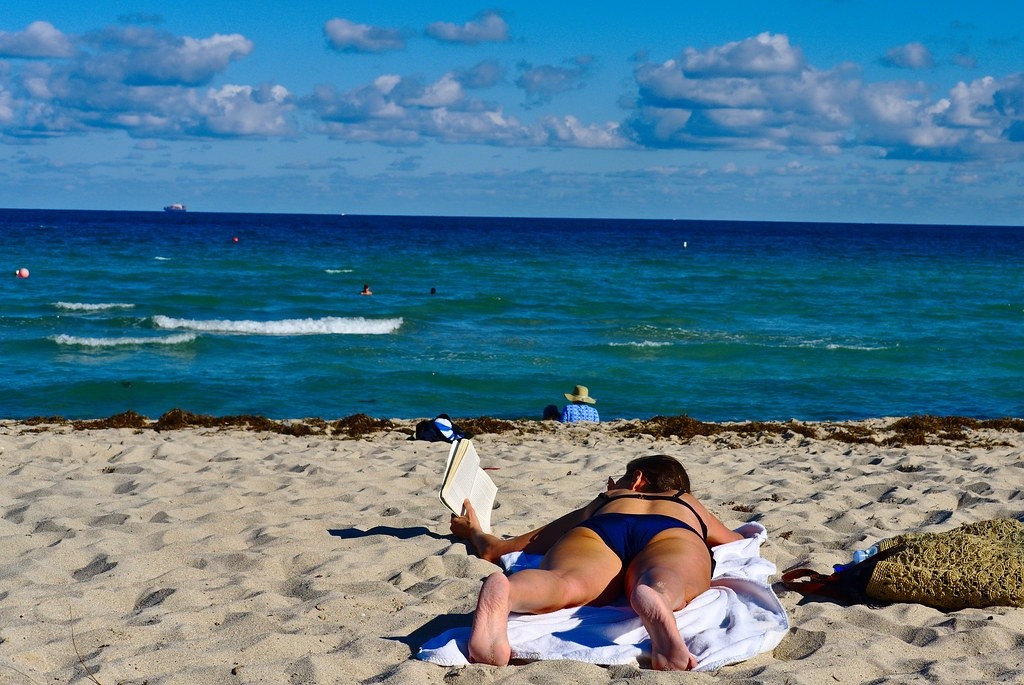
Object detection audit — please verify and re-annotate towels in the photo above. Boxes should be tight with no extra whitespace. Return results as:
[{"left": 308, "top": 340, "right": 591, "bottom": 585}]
[{"left": 416, "top": 521, "right": 791, "bottom": 671}]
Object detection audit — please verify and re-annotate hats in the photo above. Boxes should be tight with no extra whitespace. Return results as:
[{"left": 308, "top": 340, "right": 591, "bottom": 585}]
[{"left": 565, "top": 384, "right": 595, "bottom": 404}]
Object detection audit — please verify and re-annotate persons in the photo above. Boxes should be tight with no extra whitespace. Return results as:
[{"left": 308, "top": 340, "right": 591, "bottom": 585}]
[
  {"left": 359, "top": 285, "right": 375, "bottom": 295},
  {"left": 450, "top": 455, "right": 747, "bottom": 671},
  {"left": 559, "top": 385, "right": 600, "bottom": 423}
]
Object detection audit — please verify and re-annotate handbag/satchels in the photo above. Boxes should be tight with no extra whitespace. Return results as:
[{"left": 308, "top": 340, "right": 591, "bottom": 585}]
[{"left": 781, "top": 518, "right": 1024, "bottom": 610}]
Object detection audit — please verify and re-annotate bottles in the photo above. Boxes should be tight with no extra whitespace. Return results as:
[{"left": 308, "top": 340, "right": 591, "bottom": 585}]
[{"left": 853, "top": 542, "right": 879, "bottom": 564}]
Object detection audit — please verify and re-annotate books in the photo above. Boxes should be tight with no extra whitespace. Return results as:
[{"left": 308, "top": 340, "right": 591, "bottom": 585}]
[{"left": 438, "top": 439, "right": 501, "bottom": 534}]
